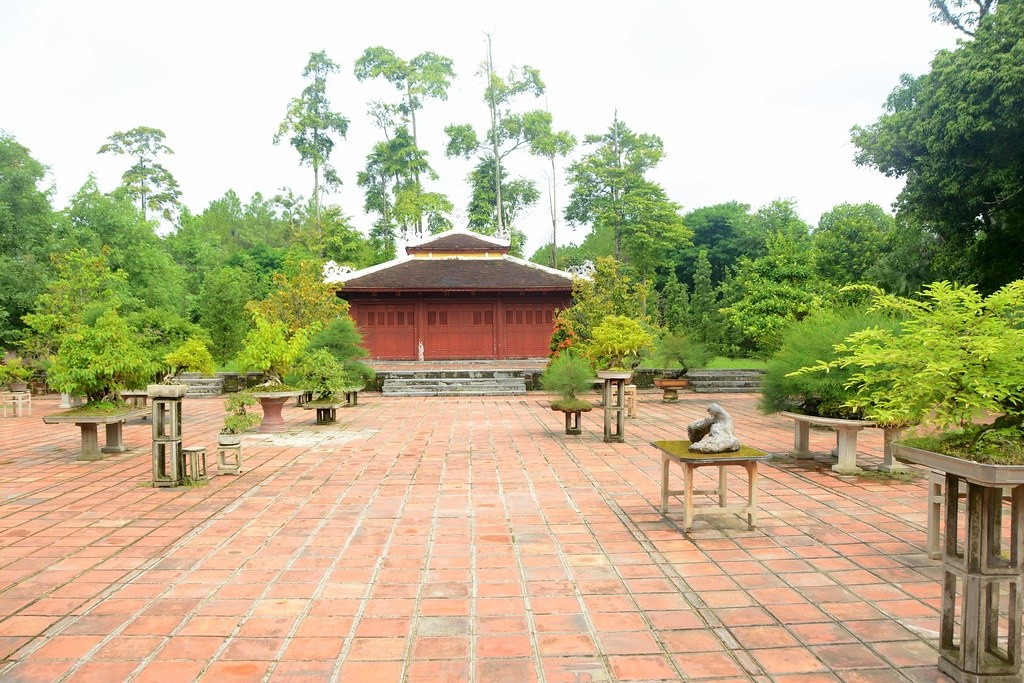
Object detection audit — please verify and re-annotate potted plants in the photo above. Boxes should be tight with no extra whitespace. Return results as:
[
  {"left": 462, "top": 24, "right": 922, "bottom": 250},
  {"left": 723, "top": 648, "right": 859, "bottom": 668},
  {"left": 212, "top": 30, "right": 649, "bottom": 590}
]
[
  {"left": 757, "top": 280, "right": 1024, "bottom": 484},
  {"left": 0, "top": 358, "right": 33, "bottom": 393},
  {"left": 19, "top": 247, "right": 372, "bottom": 433},
  {"left": 541, "top": 316, "right": 715, "bottom": 415},
  {"left": 218, "top": 391, "right": 261, "bottom": 445}
]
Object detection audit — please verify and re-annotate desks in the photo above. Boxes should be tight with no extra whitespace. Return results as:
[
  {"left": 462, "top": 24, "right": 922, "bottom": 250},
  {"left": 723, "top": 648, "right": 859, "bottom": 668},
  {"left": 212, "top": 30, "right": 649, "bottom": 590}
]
[
  {"left": 608, "top": 385, "right": 637, "bottom": 419},
  {"left": 652, "top": 439, "right": 772, "bottom": 533}
]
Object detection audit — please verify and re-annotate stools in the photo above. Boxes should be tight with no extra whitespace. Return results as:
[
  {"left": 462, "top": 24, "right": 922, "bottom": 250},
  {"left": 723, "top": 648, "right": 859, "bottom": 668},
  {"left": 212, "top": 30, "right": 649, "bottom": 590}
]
[
  {"left": 217, "top": 444, "right": 243, "bottom": 475},
  {"left": 183, "top": 445, "right": 209, "bottom": 481}
]
